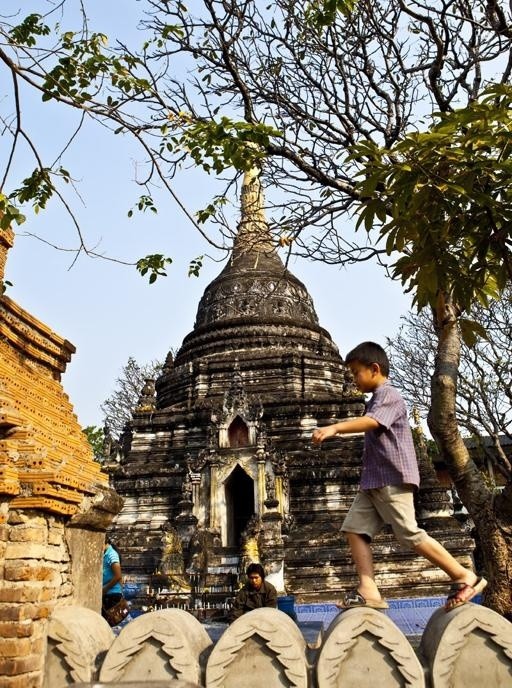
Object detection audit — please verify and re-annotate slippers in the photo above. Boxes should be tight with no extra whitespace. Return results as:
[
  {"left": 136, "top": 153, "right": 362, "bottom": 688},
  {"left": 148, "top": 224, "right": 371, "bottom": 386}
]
[
  {"left": 443, "top": 576, "right": 488, "bottom": 612},
  {"left": 336, "top": 591, "right": 390, "bottom": 610}
]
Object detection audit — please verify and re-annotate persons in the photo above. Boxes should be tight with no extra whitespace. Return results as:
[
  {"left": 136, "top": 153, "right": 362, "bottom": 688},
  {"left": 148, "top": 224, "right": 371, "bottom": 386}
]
[
  {"left": 103, "top": 534, "right": 125, "bottom": 614},
  {"left": 228, "top": 563, "right": 278, "bottom": 625},
  {"left": 310, "top": 340, "right": 488, "bottom": 612}
]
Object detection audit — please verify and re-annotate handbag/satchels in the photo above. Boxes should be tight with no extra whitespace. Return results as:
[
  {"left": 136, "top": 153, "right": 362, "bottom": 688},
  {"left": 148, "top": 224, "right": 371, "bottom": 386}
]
[{"left": 102, "top": 598, "right": 130, "bottom": 627}]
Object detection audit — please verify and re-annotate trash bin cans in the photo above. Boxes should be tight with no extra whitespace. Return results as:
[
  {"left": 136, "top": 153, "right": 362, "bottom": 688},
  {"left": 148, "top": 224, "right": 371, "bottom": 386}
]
[{"left": 277, "top": 595, "right": 297, "bottom": 621}]
